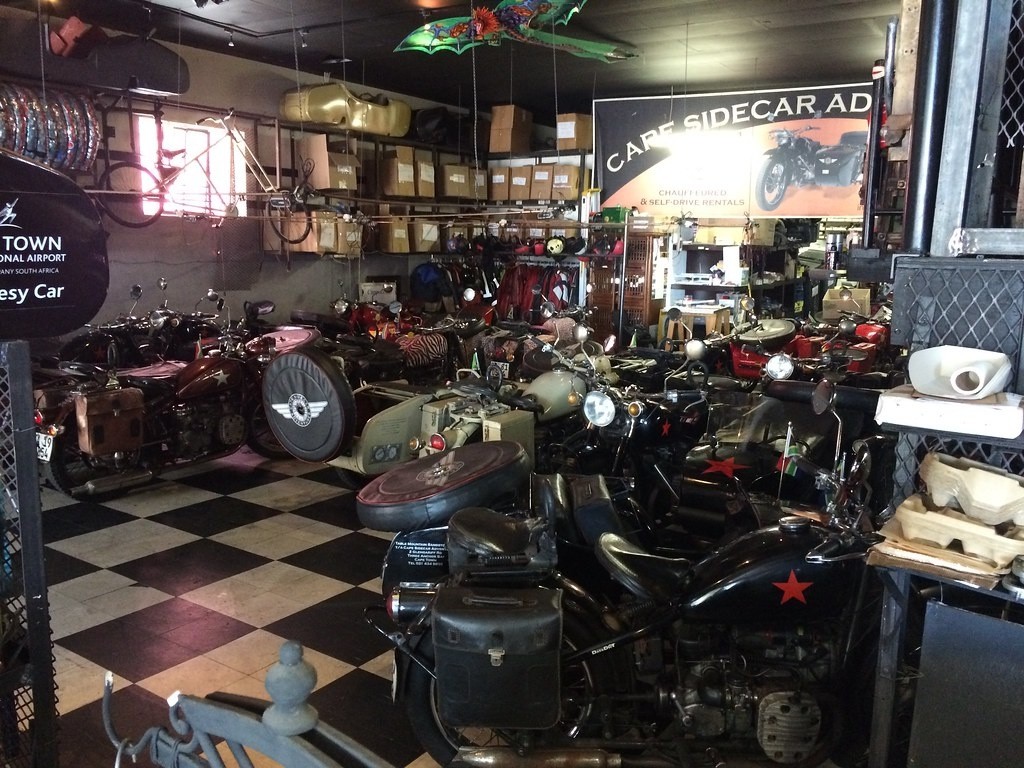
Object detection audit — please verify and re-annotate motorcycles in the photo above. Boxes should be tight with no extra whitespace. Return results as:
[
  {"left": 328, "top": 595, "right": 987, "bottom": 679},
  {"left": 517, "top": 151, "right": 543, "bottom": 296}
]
[
  {"left": 755, "top": 124, "right": 869, "bottom": 212},
  {"left": 29, "top": 277, "right": 897, "bottom": 768}
]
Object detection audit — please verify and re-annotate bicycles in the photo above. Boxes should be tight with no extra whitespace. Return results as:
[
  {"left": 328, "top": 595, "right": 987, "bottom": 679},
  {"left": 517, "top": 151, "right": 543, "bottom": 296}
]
[{"left": 99, "top": 106, "right": 312, "bottom": 244}]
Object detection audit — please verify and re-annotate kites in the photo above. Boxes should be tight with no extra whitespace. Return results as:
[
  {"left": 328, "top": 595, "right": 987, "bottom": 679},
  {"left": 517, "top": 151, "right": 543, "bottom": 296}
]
[{"left": 393, "top": 0, "right": 637, "bottom": 63}]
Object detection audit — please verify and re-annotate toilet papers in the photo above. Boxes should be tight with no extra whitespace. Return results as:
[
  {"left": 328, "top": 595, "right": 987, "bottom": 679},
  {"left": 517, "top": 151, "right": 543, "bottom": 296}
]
[{"left": 950, "top": 364, "right": 986, "bottom": 396}]
